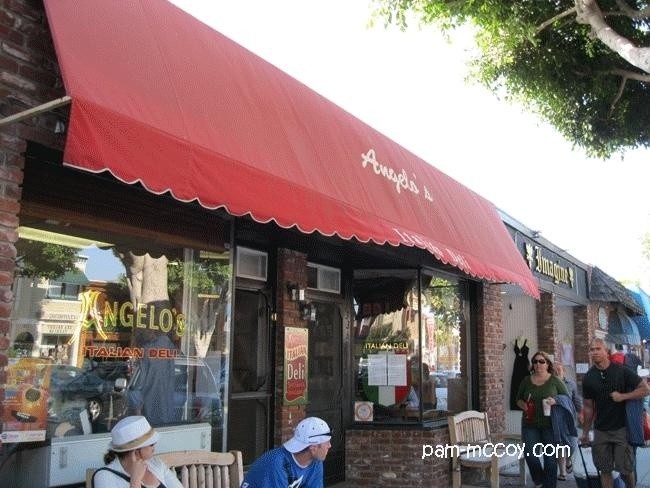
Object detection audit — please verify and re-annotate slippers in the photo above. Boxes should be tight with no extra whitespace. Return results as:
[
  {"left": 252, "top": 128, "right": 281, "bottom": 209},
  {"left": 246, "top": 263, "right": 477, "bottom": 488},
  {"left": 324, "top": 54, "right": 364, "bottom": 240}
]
[
  {"left": 566, "top": 459, "right": 573, "bottom": 474},
  {"left": 558, "top": 473, "right": 568, "bottom": 481}
]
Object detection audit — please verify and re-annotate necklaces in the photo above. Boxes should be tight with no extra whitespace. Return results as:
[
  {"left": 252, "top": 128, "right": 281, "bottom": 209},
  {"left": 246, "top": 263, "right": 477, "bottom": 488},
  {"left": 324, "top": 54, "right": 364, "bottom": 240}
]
[{"left": 593, "top": 360, "right": 610, "bottom": 380}]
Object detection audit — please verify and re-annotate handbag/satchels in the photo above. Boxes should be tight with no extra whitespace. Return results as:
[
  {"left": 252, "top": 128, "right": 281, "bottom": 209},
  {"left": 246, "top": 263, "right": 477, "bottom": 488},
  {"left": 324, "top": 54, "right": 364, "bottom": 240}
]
[{"left": 621, "top": 368, "right": 650, "bottom": 448}]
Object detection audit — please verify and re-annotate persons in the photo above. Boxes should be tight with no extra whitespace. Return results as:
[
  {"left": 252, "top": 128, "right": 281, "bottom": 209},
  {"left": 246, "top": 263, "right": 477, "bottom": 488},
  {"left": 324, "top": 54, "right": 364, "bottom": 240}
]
[
  {"left": 125, "top": 316, "right": 189, "bottom": 426},
  {"left": 517, "top": 350, "right": 570, "bottom": 488},
  {"left": 551, "top": 359, "right": 584, "bottom": 483},
  {"left": 89, "top": 414, "right": 185, "bottom": 488},
  {"left": 577, "top": 337, "right": 650, "bottom": 487},
  {"left": 239, "top": 415, "right": 332, "bottom": 488},
  {"left": 397, "top": 384, "right": 420, "bottom": 409},
  {"left": 509, "top": 335, "right": 533, "bottom": 410},
  {"left": 418, "top": 362, "right": 437, "bottom": 411}
]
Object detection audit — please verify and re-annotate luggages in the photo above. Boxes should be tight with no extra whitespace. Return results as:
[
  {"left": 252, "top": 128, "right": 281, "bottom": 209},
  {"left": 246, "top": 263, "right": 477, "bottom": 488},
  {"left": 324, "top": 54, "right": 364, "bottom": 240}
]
[{"left": 573, "top": 441, "right": 625, "bottom": 488}]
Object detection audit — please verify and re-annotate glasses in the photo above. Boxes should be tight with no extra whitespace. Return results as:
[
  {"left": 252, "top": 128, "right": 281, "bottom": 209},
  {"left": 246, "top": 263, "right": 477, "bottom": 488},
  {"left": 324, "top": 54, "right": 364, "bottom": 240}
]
[
  {"left": 309, "top": 427, "right": 336, "bottom": 439},
  {"left": 531, "top": 358, "right": 546, "bottom": 364}
]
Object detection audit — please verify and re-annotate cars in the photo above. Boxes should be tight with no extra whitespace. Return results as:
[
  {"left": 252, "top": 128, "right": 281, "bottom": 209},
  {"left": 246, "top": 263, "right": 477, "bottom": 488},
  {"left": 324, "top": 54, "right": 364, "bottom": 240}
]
[
  {"left": 430, "top": 371, "right": 461, "bottom": 388},
  {"left": 52, "top": 355, "right": 223, "bottom": 429}
]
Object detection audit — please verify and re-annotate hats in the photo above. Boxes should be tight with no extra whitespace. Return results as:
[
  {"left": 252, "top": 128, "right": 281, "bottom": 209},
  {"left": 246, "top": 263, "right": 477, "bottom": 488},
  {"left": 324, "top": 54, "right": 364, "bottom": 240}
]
[
  {"left": 281, "top": 416, "right": 332, "bottom": 454},
  {"left": 107, "top": 414, "right": 161, "bottom": 454}
]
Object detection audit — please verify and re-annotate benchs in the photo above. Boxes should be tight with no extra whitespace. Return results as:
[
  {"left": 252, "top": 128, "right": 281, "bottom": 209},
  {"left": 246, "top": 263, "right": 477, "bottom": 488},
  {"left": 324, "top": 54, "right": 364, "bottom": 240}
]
[
  {"left": 447, "top": 410, "right": 527, "bottom": 487},
  {"left": 86, "top": 450, "right": 243, "bottom": 488}
]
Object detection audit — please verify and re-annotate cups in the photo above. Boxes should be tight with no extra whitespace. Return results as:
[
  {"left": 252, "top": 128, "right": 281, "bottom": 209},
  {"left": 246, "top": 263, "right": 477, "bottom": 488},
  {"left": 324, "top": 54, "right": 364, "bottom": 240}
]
[{"left": 542, "top": 399, "right": 550, "bottom": 418}]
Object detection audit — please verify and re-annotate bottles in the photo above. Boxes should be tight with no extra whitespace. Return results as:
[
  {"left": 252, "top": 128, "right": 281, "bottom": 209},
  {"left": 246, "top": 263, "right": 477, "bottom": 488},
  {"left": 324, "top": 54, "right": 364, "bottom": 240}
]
[{"left": 523, "top": 400, "right": 535, "bottom": 425}]
